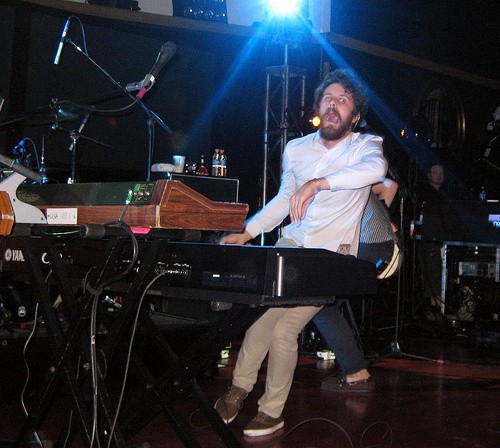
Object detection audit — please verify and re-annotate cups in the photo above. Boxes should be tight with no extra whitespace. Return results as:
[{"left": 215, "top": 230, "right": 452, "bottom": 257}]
[
  {"left": 173, "top": 156, "right": 185, "bottom": 173},
  {"left": 196, "top": 155, "right": 208, "bottom": 175}
]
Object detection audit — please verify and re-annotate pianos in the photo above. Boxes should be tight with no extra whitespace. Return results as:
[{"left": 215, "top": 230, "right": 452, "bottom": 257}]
[{"left": 0, "top": 178, "right": 377, "bottom": 448}]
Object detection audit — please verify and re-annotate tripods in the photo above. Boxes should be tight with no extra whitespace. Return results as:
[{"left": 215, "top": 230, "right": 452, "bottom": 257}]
[{"left": 367, "top": 180, "right": 452, "bottom": 365}]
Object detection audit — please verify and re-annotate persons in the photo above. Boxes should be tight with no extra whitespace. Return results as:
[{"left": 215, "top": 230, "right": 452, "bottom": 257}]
[
  {"left": 312, "top": 179, "right": 398, "bottom": 392},
  {"left": 211, "top": 69, "right": 388, "bottom": 439},
  {"left": 417, "top": 163, "right": 457, "bottom": 322}
]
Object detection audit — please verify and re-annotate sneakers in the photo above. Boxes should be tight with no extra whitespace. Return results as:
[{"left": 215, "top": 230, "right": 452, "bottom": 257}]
[
  {"left": 244, "top": 412, "right": 285, "bottom": 436},
  {"left": 214, "top": 385, "right": 250, "bottom": 424}
]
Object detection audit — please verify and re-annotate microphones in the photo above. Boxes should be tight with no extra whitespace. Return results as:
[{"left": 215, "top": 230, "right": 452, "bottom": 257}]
[
  {"left": 136, "top": 41, "right": 178, "bottom": 101},
  {"left": 52, "top": 17, "right": 71, "bottom": 65},
  {"left": 79, "top": 224, "right": 131, "bottom": 239}
]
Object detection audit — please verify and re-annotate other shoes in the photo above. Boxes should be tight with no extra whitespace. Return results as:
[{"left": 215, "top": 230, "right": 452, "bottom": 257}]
[{"left": 321, "top": 374, "right": 374, "bottom": 393}]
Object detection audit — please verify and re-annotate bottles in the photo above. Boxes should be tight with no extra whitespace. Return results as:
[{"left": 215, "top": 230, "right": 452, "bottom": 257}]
[
  {"left": 219, "top": 149, "right": 227, "bottom": 177},
  {"left": 211, "top": 149, "right": 221, "bottom": 177},
  {"left": 479, "top": 186, "right": 486, "bottom": 202}
]
